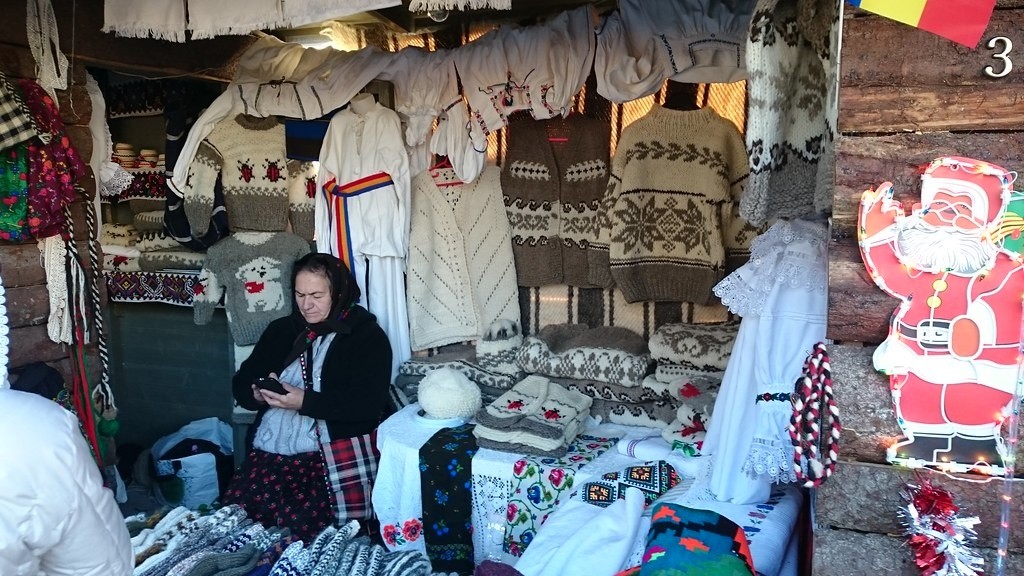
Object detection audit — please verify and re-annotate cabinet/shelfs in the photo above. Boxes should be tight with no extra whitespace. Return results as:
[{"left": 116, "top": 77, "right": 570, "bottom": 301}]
[{"left": 94, "top": 75, "right": 253, "bottom": 500}]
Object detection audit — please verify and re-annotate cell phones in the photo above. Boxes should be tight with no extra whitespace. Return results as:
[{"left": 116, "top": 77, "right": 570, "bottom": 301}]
[{"left": 253, "top": 377, "right": 291, "bottom": 395}]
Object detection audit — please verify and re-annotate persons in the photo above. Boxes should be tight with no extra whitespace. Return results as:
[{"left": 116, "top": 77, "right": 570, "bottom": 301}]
[
  {"left": 0, "top": 388, "right": 134, "bottom": 576},
  {"left": 314, "top": 92, "right": 412, "bottom": 385},
  {"left": 230, "top": 253, "right": 391, "bottom": 537}
]
